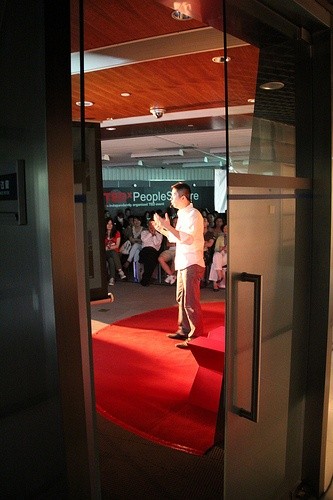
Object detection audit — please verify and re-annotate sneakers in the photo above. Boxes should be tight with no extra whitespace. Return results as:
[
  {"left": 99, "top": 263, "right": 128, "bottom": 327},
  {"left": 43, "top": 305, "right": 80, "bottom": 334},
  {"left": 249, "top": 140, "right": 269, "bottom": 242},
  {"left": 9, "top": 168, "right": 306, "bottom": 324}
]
[
  {"left": 109, "top": 278, "right": 115, "bottom": 285},
  {"left": 165, "top": 275, "right": 176, "bottom": 285},
  {"left": 118, "top": 269, "right": 126, "bottom": 279}
]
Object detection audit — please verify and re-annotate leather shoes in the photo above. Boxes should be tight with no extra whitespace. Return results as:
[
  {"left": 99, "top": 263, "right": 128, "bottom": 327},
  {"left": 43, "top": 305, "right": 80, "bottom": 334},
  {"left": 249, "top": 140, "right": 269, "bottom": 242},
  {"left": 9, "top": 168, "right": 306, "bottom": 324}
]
[
  {"left": 175, "top": 338, "right": 191, "bottom": 349},
  {"left": 167, "top": 334, "right": 187, "bottom": 340}
]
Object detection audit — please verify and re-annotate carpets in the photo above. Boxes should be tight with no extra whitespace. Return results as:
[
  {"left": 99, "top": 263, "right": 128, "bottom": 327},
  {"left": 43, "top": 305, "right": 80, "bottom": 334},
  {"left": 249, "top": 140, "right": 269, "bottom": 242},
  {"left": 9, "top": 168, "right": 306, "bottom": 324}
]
[{"left": 91, "top": 300, "right": 226, "bottom": 456}]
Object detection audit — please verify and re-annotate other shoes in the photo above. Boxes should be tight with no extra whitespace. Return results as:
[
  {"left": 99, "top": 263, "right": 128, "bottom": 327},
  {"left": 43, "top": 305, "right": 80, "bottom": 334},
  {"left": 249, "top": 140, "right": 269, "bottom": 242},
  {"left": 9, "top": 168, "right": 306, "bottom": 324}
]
[
  {"left": 213, "top": 278, "right": 225, "bottom": 290},
  {"left": 124, "top": 261, "right": 130, "bottom": 268}
]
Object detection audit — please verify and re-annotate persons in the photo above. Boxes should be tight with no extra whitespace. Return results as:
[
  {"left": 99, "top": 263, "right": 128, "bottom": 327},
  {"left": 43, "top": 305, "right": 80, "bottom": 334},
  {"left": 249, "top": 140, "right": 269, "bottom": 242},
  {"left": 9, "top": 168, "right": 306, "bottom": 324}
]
[
  {"left": 105, "top": 208, "right": 227, "bottom": 290},
  {"left": 150, "top": 182, "right": 205, "bottom": 349}
]
